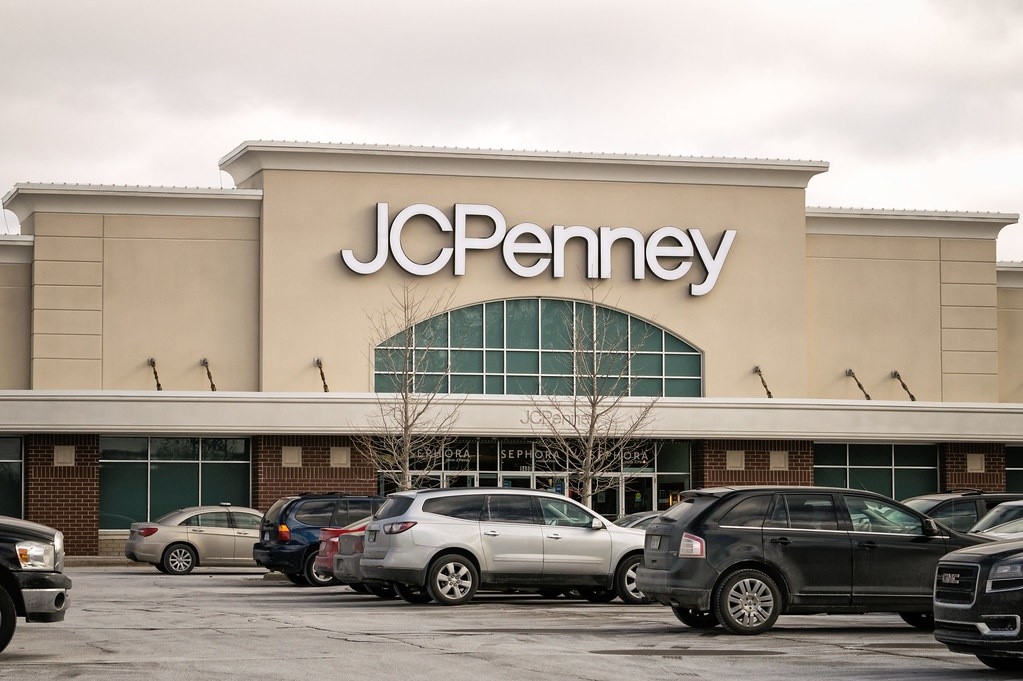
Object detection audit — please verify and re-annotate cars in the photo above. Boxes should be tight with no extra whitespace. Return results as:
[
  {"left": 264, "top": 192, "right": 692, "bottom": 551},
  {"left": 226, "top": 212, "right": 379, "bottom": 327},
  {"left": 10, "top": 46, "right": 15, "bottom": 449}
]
[
  {"left": 124, "top": 502, "right": 264, "bottom": 575},
  {"left": 965, "top": 501, "right": 1023, "bottom": 536},
  {"left": 612, "top": 511, "right": 664, "bottom": 530},
  {"left": 314, "top": 515, "right": 399, "bottom": 598}
]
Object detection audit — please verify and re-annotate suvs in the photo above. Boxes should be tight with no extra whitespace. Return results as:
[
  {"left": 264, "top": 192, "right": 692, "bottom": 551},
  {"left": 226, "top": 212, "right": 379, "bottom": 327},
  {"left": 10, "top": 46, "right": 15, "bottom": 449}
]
[
  {"left": 933, "top": 536, "right": 1023, "bottom": 672},
  {"left": 0, "top": 515, "right": 72, "bottom": 654},
  {"left": 359, "top": 486, "right": 659, "bottom": 606},
  {"left": 253, "top": 490, "right": 388, "bottom": 588},
  {"left": 635, "top": 484, "right": 1011, "bottom": 635},
  {"left": 877, "top": 487, "right": 1023, "bottom": 538}
]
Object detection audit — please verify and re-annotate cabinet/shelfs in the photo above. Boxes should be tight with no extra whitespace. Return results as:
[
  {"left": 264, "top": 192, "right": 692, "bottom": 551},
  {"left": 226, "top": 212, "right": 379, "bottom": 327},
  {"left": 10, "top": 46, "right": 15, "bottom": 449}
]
[{"left": 657, "top": 489, "right": 681, "bottom": 511}]
